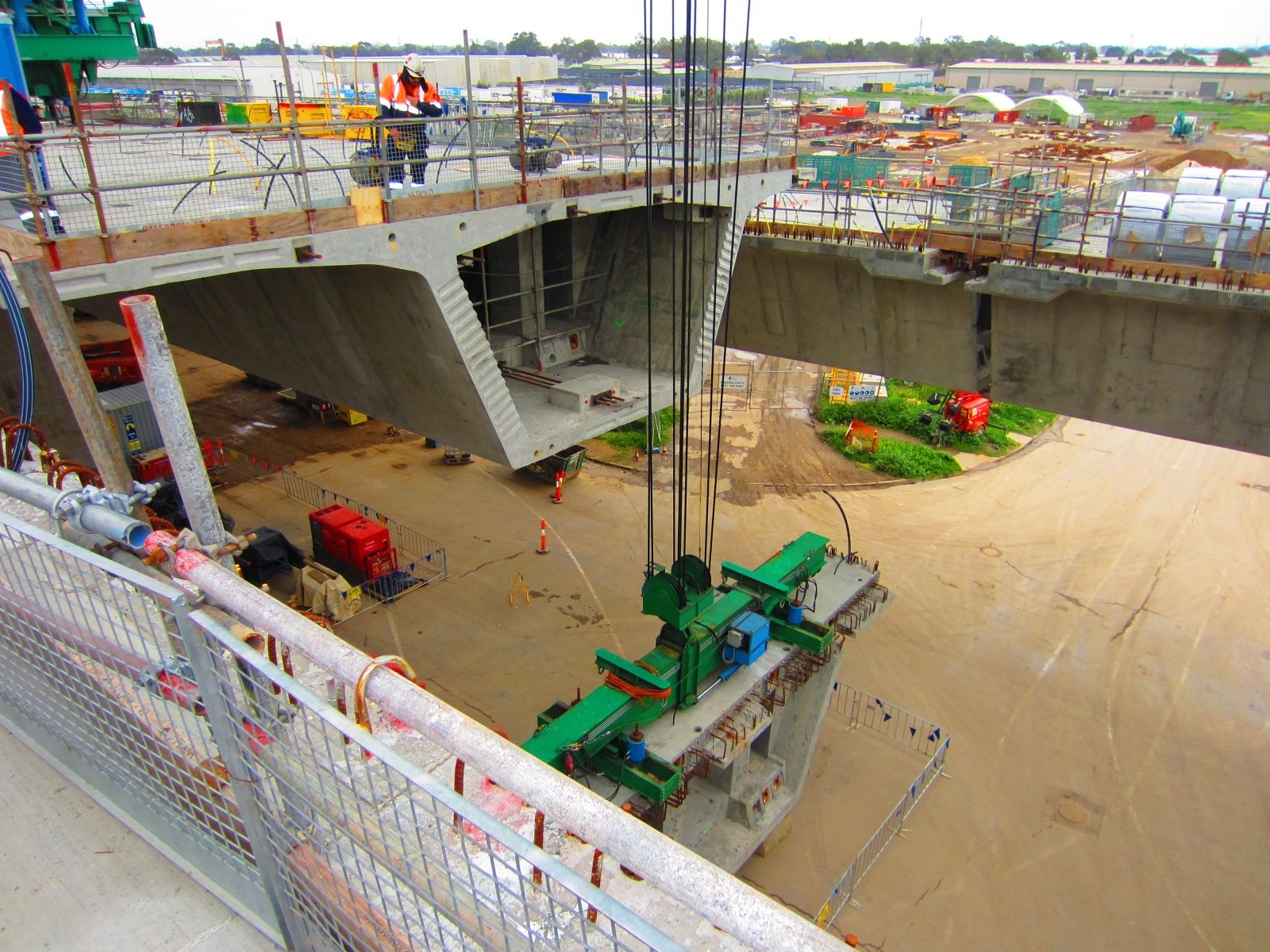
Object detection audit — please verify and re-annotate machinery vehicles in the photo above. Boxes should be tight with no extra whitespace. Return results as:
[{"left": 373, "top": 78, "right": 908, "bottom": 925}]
[
  {"left": 932, "top": 107, "right": 960, "bottom": 130},
  {"left": 944, "top": 391, "right": 989, "bottom": 434}
]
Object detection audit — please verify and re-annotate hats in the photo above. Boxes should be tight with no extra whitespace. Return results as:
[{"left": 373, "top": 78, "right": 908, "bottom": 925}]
[{"left": 406, "top": 68, "right": 424, "bottom": 78}]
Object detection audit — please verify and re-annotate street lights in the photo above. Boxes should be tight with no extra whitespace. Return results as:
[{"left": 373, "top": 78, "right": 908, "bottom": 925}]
[{"left": 1039, "top": 98, "right": 1057, "bottom": 164}]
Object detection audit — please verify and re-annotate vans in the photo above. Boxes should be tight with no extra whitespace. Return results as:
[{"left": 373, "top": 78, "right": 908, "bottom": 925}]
[{"left": 902, "top": 114, "right": 920, "bottom": 123}]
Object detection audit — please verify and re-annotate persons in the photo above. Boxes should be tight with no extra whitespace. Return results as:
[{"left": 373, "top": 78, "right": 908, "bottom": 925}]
[
  {"left": 0, "top": 79, "right": 66, "bottom": 242},
  {"left": 377, "top": 53, "right": 443, "bottom": 189}
]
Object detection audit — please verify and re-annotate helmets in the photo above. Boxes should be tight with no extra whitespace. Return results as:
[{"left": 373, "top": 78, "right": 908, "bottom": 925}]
[{"left": 404, "top": 53, "right": 425, "bottom": 76}]
